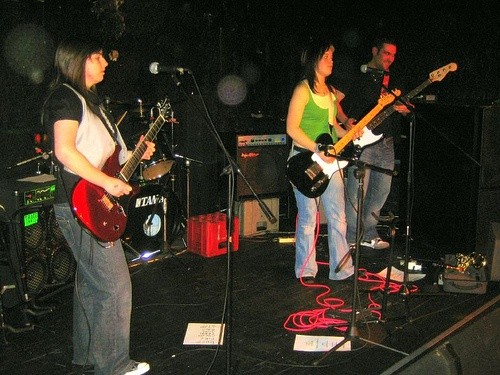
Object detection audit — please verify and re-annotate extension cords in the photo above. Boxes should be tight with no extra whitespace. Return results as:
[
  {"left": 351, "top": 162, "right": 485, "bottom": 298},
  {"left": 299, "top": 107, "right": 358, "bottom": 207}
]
[{"left": 277, "top": 236, "right": 295, "bottom": 243}]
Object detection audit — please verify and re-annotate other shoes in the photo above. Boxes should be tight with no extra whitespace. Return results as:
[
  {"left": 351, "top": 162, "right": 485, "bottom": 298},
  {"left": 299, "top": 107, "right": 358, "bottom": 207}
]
[
  {"left": 346, "top": 275, "right": 354, "bottom": 281},
  {"left": 125, "top": 362, "right": 150, "bottom": 375},
  {"left": 349, "top": 243, "right": 356, "bottom": 249},
  {"left": 303, "top": 277, "right": 315, "bottom": 284},
  {"left": 360, "top": 238, "right": 389, "bottom": 249}
]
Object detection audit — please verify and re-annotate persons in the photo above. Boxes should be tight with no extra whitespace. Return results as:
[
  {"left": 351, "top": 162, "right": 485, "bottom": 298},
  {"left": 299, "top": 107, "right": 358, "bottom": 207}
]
[
  {"left": 285, "top": 36, "right": 357, "bottom": 285},
  {"left": 43, "top": 33, "right": 156, "bottom": 375},
  {"left": 344, "top": 38, "right": 397, "bottom": 248}
]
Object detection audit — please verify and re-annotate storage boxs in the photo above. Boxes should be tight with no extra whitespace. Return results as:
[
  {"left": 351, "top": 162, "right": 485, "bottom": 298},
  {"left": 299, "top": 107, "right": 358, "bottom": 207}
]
[
  {"left": 186, "top": 212, "right": 239, "bottom": 258},
  {"left": 233, "top": 197, "right": 279, "bottom": 238}
]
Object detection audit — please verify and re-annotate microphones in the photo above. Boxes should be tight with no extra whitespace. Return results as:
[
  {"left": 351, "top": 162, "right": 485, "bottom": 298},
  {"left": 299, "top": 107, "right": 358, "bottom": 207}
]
[
  {"left": 360, "top": 66, "right": 388, "bottom": 75},
  {"left": 148, "top": 62, "right": 191, "bottom": 75},
  {"left": 314, "top": 142, "right": 334, "bottom": 152}
]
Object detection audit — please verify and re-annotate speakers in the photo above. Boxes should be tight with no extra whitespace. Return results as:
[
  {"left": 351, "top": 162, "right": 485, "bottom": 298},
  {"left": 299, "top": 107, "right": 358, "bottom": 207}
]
[
  {"left": 169, "top": 95, "right": 218, "bottom": 224},
  {"left": 0, "top": 205, "right": 77, "bottom": 311},
  {"left": 119, "top": 183, "right": 168, "bottom": 268},
  {"left": 237, "top": 145, "right": 288, "bottom": 196}
]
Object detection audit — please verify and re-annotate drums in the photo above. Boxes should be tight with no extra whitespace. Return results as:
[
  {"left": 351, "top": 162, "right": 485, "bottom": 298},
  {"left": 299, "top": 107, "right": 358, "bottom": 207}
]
[
  {"left": 109, "top": 102, "right": 148, "bottom": 142},
  {"left": 125, "top": 127, "right": 177, "bottom": 182},
  {"left": 118, "top": 183, "right": 182, "bottom": 251}
]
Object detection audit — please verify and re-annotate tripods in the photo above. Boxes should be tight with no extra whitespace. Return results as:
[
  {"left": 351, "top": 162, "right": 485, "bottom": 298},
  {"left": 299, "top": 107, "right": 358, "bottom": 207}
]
[{"left": 312, "top": 76, "right": 482, "bottom": 367}]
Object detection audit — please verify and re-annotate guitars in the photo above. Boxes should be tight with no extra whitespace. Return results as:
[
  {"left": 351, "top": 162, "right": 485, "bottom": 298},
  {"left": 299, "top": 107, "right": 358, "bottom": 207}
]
[
  {"left": 285, "top": 89, "right": 401, "bottom": 199},
  {"left": 71, "top": 98, "right": 174, "bottom": 241},
  {"left": 337, "top": 62, "right": 457, "bottom": 167}
]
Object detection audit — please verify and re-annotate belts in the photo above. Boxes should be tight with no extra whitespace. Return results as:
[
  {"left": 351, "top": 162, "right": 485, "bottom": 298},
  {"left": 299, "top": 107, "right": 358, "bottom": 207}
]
[{"left": 293, "top": 145, "right": 309, "bottom": 153}]
